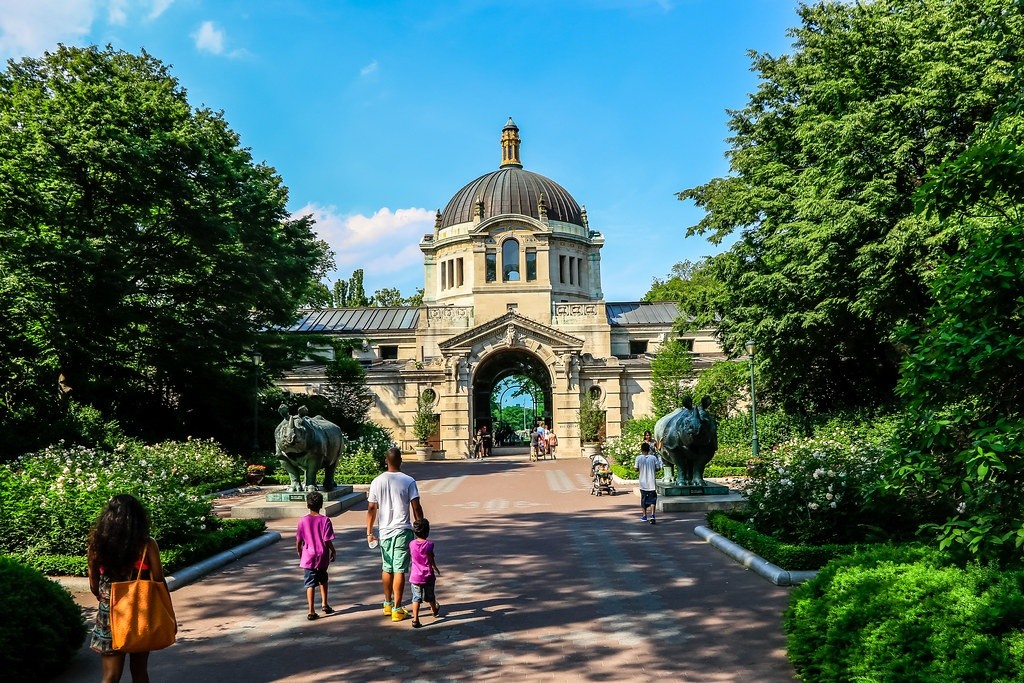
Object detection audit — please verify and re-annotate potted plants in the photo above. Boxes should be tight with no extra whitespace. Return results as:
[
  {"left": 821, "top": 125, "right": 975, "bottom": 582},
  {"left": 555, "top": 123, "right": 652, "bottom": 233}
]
[
  {"left": 246, "top": 464, "right": 267, "bottom": 484},
  {"left": 576, "top": 390, "right": 608, "bottom": 457},
  {"left": 410, "top": 389, "right": 440, "bottom": 461}
]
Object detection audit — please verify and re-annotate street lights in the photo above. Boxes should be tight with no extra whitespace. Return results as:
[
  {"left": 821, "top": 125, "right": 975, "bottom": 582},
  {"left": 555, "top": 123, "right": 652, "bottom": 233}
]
[
  {"left": 745, "top": 337, "right": 759, "bottom": 456},
  {"left": 251, "top": 350, "right": 263, "bottom": 450}
]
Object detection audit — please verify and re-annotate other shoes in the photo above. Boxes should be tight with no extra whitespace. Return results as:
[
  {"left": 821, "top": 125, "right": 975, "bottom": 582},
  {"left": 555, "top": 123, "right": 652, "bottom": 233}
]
[
  {"left": 432, "top": 601, "right": 440, "bottom": 617},
  {"left": 412, "top": 620, "right": 420, "bottom": 628},
  {"left": 308, "top": 612, "right": 319, "bottom": 620},
  {"left": 321, "top": 605, "right": 334, "bottom": 614}
]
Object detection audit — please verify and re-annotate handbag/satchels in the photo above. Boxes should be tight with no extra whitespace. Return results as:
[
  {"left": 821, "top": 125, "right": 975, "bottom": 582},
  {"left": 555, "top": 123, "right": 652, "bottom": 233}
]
[
  {"left": 110, "top": 539, "right": 176, "bottom": 653},
  {"left": 654, "top": 453, "right": 660, "bottom": 472}
]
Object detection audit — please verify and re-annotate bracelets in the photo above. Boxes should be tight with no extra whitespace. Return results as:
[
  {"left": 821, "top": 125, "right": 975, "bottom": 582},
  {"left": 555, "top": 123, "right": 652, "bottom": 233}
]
[{"left": 367, "top": 533, "right": 374, "bottom": 536}]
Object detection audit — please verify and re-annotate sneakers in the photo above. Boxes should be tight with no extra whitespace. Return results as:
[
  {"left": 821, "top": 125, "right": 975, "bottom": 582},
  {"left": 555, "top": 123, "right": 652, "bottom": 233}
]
[
  {"left": 391, "top": 606, "right": 411, "bottom": 621},
  {"left": 640, "top": 514, "right": 647, "bottom": 522},
  {"left": 649, "top": 515, "right": 655, "bottom": 524},
  {"left": 383, "top": 598, "right": 394, "bottom": 615}
]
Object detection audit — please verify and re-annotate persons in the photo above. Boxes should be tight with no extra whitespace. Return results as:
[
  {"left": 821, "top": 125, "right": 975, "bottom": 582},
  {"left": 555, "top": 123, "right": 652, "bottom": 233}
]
[
  {"left": 530, "top": 421, "right": 557, "bottom": 461},
  {"left": 599, "top": 464, "right": 609, "bottom": 484},
  {"left": 409, "top": 518, "right": 440, "bottom": 627},
  {"left": 634, "top": 443, "right": 661, "bottom": 524},
  {"left": 87, "top": 494, "right": 177, "bottom": 683},
  {"left": 473, "top": 426, "right": 492, "bottom": 460},
  {"left": 643, "top": 431, "right": 661, "bottom": 454},
  {"left": 367, "top": 448, "right": 424, "bottom": 621},
  {"left": 296, "top": 492, "right": 336, "bottom": 620}
]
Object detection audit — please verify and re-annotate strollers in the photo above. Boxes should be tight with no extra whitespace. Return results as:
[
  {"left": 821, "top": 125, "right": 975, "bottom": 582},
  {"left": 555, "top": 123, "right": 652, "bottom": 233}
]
[{"left": 589, "top": 453, "right": 615, "bottom": 496}]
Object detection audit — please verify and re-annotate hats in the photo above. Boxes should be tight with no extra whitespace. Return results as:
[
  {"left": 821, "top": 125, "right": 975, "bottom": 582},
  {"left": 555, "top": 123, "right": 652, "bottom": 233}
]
[{"left": 483, "top": 426, "right": 487, "bottom": 429}]
